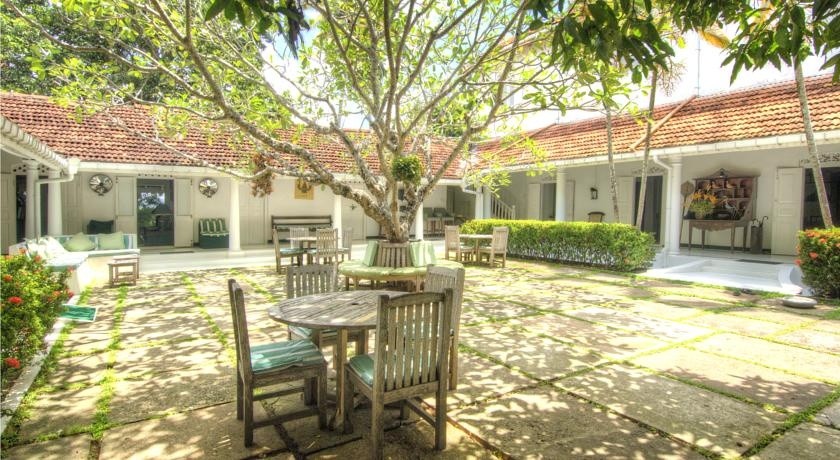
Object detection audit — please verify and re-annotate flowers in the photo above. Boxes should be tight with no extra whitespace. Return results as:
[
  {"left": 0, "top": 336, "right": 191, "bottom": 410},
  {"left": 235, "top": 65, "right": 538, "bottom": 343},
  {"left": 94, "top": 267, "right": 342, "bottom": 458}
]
[{"left": 689, "top": 190, "right": 719, "bottom": 213}]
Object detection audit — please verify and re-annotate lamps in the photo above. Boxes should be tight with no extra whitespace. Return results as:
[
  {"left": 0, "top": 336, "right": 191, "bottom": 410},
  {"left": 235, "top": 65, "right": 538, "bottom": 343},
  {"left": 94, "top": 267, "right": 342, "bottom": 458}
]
[
  {"left": 198, "top": 178, "right": 219, "bottom": 199},
  {"left": 591, "top": 188, "right": 597, "bottom": 199},
  {"left": 89, "top": 174, "right": 114, "bottom": 196}
]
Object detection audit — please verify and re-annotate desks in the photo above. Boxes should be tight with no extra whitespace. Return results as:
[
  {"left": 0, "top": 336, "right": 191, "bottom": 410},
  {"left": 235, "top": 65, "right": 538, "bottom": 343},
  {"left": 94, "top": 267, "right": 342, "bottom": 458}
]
[
  {"left": 459, "top": 234, "right": 492, "bottom": 265},
  {"left": 268, "top": 290, "right": 432, "bottom": 431},
  {"left": 286, "top": 237, "right": 331, "bottom": 264},
  {"left": 108, "top": 254, "right": 139, "bottom": 287}
]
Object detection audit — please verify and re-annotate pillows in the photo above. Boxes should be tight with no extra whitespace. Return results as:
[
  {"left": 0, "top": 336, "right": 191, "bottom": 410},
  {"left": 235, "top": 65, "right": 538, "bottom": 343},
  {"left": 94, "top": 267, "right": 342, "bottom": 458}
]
[
  {"left": 98, "top": 231, "right": 125, "bottom": 250},
  {"left": 27, "top": 236, "right": 64, "bottom": 260},
  {"left": 63, "top": 232, "right": 95, "bottom": 252}
]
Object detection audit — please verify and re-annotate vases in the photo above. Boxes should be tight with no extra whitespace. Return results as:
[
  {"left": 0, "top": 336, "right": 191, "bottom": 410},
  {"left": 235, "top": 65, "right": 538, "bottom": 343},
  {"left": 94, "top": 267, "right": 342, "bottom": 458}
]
[{"left": 695, "top": 213, "right": 705, "bottom": 219}]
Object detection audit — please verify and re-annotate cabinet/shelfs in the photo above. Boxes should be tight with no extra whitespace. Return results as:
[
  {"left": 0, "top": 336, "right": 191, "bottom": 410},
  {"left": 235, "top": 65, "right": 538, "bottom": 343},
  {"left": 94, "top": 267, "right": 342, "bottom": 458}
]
[{"left": 683, "top": 168, "right": 761, "bottom": 253}]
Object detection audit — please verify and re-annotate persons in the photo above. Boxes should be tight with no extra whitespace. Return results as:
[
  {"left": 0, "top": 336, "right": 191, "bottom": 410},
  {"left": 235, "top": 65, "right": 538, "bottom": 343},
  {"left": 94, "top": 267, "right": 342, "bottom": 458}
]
[{"left": 157, "top": 187, "right": 165, "bottom": 205}]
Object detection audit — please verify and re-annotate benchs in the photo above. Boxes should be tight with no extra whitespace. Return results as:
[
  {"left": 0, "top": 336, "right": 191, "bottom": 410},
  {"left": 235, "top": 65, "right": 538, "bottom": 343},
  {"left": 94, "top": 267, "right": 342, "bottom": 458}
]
[
  {"left": 268, "top": 215, "right": 333, "bottom": 244},
  {"left": 9, "top": 233, "right": 140, "bottom": 293},
  {"left": 199, "top": 218, "right": 229, "bottom": 249},
  {"left": 338, "top": 239, "right": 464, "bottom": 293}
]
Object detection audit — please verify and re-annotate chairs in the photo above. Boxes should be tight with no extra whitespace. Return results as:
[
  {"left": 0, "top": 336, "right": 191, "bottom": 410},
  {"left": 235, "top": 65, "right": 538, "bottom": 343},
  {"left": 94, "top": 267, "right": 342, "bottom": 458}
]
[
  {"left": 477, "top": 226, "right": 509, "bottom": 268},
  {"left": 228, "top": 226, "right": 473, "bottom": 460},
  {"left": 423, "top": 207, "right": 454, "bottom": 238},
  {"left": 588, "top": 212, "right": 605, "bottom": 222}
]
